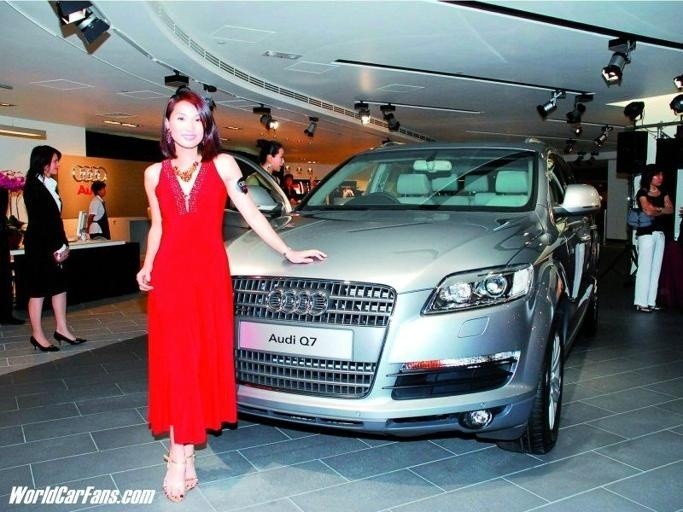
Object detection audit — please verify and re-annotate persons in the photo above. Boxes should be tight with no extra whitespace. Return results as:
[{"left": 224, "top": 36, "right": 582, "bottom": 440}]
[
  {"left": 243, "top": 139, "right": 285, "bottom": 206},
  {"left": 281, "top": 173, "right": 299, "bottom": 205},
  {"left": 22, "top": 144, "right": 87, "bottom": 353},
  {"left": 85, "top": 180, "right": 112, "bottom": 242},
  {"left": 633, "top": 164, "right": 674, "bottom": 314},
  {"left": 135, "top": 90, "right": 327, "bottom": 503}
]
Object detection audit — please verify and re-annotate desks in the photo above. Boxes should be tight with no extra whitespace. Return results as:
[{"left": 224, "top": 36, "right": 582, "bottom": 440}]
[{"left": 7, "top": 238, "right": 142, "bottom": 320}]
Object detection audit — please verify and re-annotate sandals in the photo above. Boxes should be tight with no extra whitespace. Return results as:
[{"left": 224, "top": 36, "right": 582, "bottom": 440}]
[{"left": 164, "top": 452, "right": 198, "bottom": 503}]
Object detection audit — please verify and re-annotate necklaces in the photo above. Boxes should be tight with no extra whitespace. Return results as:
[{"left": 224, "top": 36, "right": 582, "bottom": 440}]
[
  {"left": 649, "top": 188, "right": 659, "bottom": 193},
  {"left": 171, "top": 155, "right": 202, "bottom": 182}
]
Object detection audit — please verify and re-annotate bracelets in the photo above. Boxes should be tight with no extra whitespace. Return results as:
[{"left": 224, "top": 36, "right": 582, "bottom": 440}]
[{"left": 283, "top": 246, "right": 292, "bottom": 257}]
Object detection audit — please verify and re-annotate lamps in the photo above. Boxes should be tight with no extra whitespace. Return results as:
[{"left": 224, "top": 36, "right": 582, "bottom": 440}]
[
  {"left": 0, "top": 110, "right": 47, "bottom": 141},
  {"left": 534, "top": 34, "right": 682, "bottom": 166},
  {"left": 57, "top": 0, "right": 400, "bottom": 140}
]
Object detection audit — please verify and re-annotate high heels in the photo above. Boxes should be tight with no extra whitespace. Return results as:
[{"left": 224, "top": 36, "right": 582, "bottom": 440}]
[
  {"left": 30, "top": 336, "right": 59, "bottom": 351},
  {"left": 54, "top": 331, "right": 87, "bottom": 345}
]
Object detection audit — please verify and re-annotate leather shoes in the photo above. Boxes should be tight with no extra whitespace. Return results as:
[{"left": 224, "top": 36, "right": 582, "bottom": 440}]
[{"left": 637, "top": 305, "right": 660, "bottom": 312}]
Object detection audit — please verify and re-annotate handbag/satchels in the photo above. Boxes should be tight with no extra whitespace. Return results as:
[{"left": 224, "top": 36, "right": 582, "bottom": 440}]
[{"left": 627, "top": 208, "right": 655, "bottom": 228}]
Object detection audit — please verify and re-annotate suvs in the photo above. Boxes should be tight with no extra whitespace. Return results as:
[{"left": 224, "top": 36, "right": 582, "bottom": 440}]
[{"left": 223, "top": 139, "right": 603, "bottom": 456}]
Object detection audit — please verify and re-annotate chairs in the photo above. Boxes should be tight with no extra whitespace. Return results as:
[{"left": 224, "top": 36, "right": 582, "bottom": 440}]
[{"left": 386, "top": 166, "right": 530, "bottom": 209}]
